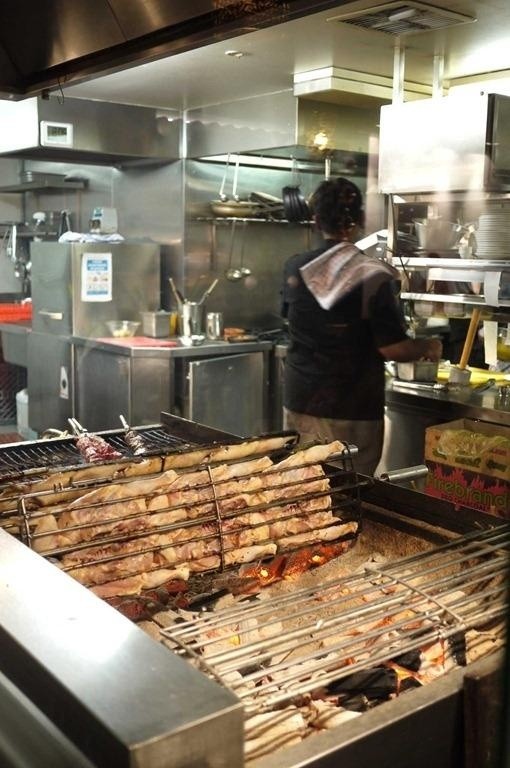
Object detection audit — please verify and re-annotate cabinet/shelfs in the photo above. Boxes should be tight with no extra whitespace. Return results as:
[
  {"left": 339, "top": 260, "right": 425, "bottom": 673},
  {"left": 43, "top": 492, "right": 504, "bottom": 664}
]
[{"left": 371, "top": 195, "right": 509, "bottom": 412}]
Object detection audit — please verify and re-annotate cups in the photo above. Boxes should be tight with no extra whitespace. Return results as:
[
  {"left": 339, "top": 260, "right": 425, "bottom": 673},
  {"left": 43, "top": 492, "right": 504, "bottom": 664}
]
[{"left": 205, "top": 312, "right": 225, "bottom": 341}]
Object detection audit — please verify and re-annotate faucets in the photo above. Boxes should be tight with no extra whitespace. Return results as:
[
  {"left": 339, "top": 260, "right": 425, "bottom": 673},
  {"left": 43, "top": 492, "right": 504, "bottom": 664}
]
[{"left": 21, "top": 297, "right": 32, "bottom": 305}]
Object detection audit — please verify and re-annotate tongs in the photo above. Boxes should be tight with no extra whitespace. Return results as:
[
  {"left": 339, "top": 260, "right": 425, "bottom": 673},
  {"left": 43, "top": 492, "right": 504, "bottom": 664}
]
[{"left": 391, "top": 379, "right": 449, "bottom": 393}]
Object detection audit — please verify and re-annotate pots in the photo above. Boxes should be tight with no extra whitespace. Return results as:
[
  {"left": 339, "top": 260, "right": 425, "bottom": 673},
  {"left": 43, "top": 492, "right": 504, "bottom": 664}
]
[{"left": 282, "top": 154, "right": 312, "bottom": 224}]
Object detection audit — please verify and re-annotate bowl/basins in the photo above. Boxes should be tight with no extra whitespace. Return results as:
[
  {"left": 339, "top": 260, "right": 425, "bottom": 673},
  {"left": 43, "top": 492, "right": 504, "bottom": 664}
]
[
  {"left": 223, "top": 328, "right": 245, "bottom": 340},
  {"left": 178, "top": 334, "right": 205, "bottom": 347},
  {"left": 410, "top": 217, "right": 469, "bottom": 250},
  {"left": 211, "top": 199, "right": 260, "bottom": 217},
  {"left": 106, "top": 320, "right": 141, "bottom": 338}
]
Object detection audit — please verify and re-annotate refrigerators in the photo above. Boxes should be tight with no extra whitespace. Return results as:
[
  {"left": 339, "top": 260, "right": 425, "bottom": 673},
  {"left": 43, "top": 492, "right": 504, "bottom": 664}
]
[{"left": 29, "top": 240, "right": 162, "bottom": 438}]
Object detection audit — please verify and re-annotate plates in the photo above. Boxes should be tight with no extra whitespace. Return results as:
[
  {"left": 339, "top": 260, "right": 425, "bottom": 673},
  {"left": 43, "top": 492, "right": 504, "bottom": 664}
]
[{"left": 474, "top": 215, "right": 510, "bottom": 259}]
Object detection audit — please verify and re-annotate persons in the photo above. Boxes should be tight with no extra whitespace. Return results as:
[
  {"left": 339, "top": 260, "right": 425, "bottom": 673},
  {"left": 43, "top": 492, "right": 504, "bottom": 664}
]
[{"left": 281, "top": 177, "right": 442, "bottom": 478}]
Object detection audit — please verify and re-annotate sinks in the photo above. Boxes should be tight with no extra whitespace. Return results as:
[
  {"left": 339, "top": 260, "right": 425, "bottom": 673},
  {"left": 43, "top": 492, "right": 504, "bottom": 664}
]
[{"left": 1, "top": 318, "right": 32, "bottom": 369}]
